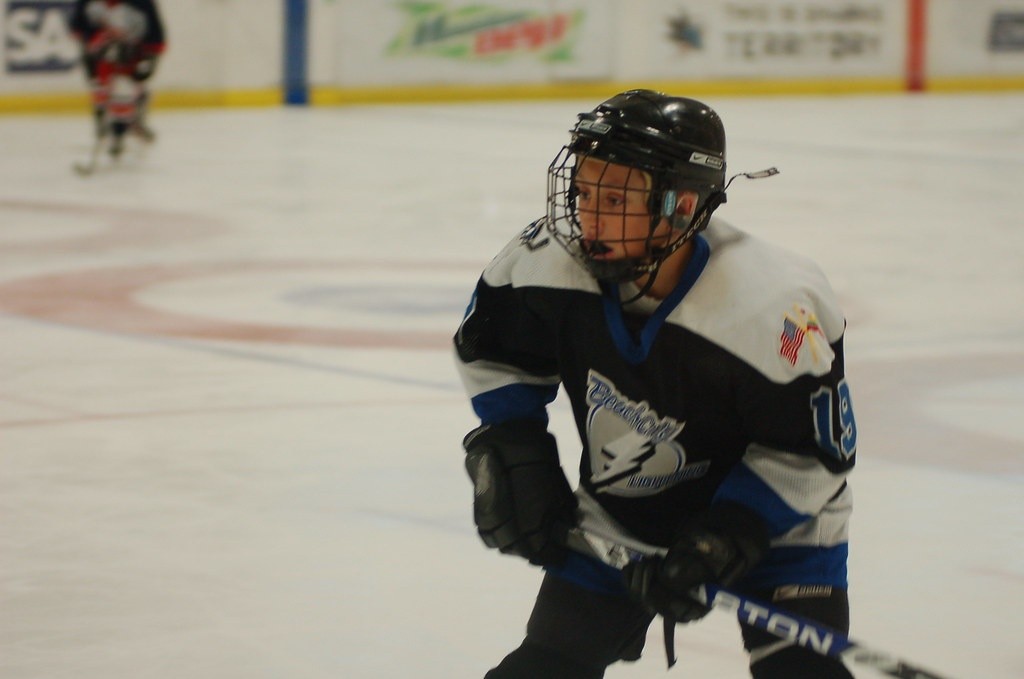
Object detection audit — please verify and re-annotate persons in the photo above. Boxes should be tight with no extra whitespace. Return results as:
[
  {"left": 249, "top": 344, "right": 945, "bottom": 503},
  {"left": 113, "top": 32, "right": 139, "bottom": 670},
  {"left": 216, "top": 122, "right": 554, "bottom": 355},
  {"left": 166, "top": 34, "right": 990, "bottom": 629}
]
[
  {"left": 67, "top": 0, "right": 168, "bottom": 165},
  {"left": 450, "top": 88, "right": 855, "bottom": 679}
]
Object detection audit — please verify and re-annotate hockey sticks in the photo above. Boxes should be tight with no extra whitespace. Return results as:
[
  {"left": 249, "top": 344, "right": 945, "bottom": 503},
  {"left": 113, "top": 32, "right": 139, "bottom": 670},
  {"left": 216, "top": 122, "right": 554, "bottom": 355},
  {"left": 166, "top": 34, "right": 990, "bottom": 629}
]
[
  {"left": 565, "top": 524, "right": 944, "bottom": 677},
  {"left": 70, "top": 130, "right": 105, "bottom": 176}
]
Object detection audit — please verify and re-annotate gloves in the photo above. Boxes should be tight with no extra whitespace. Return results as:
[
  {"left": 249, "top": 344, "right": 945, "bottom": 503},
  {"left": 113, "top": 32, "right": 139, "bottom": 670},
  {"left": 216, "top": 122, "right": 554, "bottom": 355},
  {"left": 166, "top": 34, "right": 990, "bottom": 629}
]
[
  {"left": 623, "top": 499, "right": 772, "bottom": 624},
  {"left": 463, "top": 417, "right": 580, "bottom": 566}
]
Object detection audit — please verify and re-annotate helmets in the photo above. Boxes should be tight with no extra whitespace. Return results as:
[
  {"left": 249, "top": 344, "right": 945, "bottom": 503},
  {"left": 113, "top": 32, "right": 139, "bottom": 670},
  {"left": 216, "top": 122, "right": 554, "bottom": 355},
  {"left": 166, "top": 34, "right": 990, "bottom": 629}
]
[{"left": 546, "top": 89, "right": 727, "bottom": 282}]
